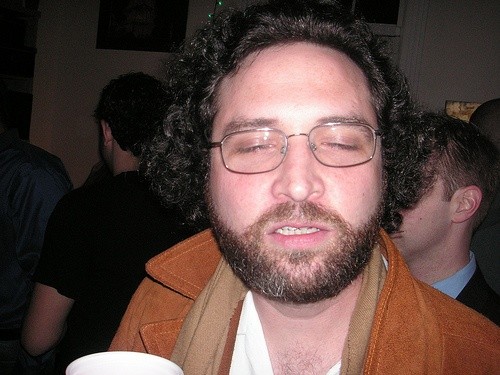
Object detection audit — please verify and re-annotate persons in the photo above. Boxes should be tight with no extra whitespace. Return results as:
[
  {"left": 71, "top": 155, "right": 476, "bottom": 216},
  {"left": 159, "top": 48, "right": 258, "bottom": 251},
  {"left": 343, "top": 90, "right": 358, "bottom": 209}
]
[
  {"left": 107, "top": 0, "right": 500, "bottom": 374},
  {"left": 379, "top": 99, "right": 500, "bottom": 327},
  {"left": 0, "top": 78, "right": 72, "bottom": 375},
  {"left": 22, "top": 73, "right": 196, "bottom": 375}
]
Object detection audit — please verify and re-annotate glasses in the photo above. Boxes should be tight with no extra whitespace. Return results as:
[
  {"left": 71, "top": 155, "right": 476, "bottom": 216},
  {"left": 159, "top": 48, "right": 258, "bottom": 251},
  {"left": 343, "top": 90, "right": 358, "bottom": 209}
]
[{"left": 205, "top": 121, "right": 383, "bottom": 174}]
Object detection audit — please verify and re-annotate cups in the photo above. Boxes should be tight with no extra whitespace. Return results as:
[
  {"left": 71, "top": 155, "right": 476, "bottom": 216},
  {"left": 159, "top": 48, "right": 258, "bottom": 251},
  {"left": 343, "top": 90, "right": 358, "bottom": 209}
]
[{"left": 65, "top": 351, "right": 185, "bottom": 375}]
